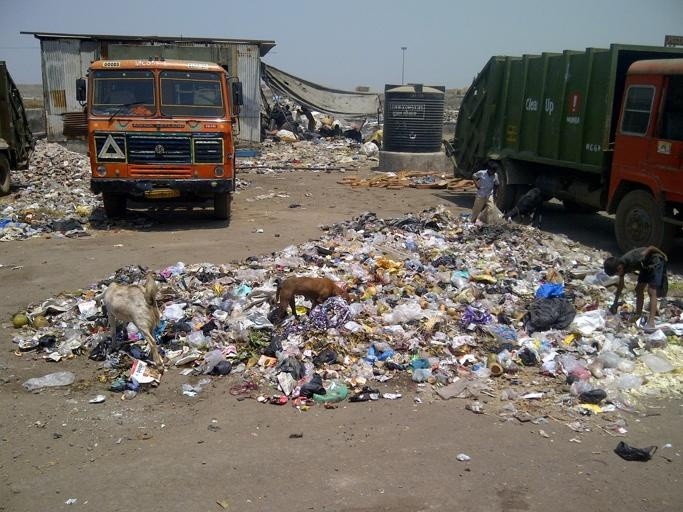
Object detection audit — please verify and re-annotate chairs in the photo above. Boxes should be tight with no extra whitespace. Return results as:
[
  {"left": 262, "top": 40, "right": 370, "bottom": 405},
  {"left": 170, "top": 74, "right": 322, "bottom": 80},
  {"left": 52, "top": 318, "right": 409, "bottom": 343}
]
[
  {"left": 109, "top": 91, "right": 135, "bottom": 103},
  {"left": 193, "top": 89, "right": 216, "bottom": 105}
]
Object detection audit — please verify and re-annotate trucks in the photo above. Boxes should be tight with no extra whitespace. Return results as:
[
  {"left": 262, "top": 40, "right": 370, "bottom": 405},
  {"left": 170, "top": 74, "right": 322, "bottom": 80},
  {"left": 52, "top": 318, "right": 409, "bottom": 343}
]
[
  {"left": 75, "top": 56, "right": 244, "bottom": 222},
  {"left": 440, "top": 42, "right": 683, "bottom": 257}
]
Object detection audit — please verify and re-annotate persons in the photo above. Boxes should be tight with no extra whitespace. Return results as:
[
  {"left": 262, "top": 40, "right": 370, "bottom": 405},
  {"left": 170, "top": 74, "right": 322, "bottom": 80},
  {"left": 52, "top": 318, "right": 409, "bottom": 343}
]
[
  {"left": 604, "top": 245, "right": 668, "bottom": 328},
  {"left": 471, "top": 163, "right": 500, "bottom": 223}
]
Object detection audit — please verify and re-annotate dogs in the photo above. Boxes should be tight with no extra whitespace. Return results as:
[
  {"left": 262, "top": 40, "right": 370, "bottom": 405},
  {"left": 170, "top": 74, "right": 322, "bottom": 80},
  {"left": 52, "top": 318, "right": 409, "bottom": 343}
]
[
  {"left": 269, "top": 276, "right": 358, "bottom": 319},
  {"left": 103, "top": 272, "right": 166, "bottom": 376}
]
